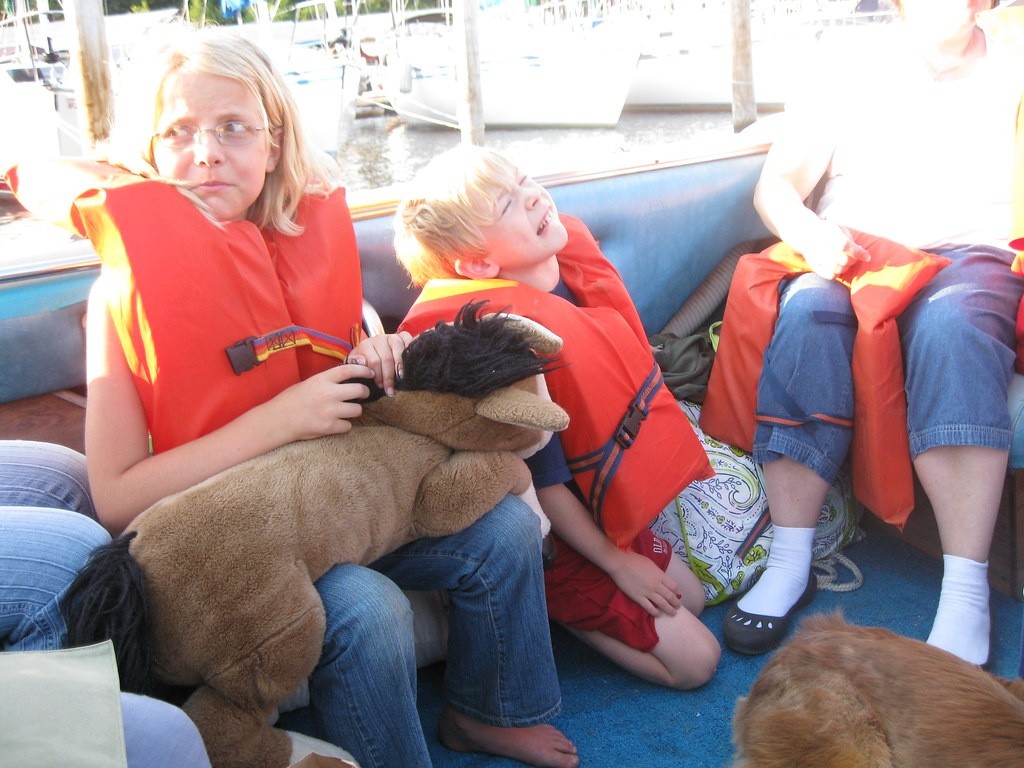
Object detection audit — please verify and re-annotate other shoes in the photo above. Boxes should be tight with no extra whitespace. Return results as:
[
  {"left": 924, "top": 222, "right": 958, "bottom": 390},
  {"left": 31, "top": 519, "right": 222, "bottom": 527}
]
[
  {"left": 724, "top": 567, "right": 818, "bottom": 655},
  {"left": 976, "top": 601, "right": 995, "bottom": 673}
]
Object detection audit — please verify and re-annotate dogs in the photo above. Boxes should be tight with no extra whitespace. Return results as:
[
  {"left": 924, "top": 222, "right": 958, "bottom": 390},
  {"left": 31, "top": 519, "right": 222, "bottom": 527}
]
[{"left": 728, "top": 604, "right": 1024, "bottom": 767}]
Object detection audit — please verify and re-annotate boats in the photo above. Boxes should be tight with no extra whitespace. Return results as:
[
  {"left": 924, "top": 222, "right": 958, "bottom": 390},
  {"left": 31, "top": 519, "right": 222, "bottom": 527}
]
[{"left": 1, "top": 0, "right": 908, "bottom": 163}]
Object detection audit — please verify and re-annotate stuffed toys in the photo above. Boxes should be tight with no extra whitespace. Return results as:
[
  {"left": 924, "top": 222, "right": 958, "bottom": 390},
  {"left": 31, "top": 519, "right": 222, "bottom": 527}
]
[{"left": 60, "top": 299, "right": 570, "bottom": 768}]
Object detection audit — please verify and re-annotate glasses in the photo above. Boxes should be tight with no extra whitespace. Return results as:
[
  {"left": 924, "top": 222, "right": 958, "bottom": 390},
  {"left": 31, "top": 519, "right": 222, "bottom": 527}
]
[{"left": 152, "top": 122, "right": 265, "bottom": 147}]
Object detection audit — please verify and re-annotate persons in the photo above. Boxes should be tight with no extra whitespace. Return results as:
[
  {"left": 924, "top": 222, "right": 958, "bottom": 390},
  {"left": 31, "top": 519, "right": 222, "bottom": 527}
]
[
  {"left": 71, "top": 33, "right": 578, "bottom": 768},
  {"left": 391, "top": 143, "right": 721, "bottom": 692},
  {"left": 0, "top": 437, "right": 113, "bottom": 652},
  {"left": 726, "top": 0, "right": 1024, "bottom": 674}
]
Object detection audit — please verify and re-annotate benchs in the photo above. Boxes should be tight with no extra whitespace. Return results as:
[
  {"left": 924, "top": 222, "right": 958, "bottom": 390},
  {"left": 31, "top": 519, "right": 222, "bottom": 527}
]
[{"left": 0, "top": 149, "right": 1024, "bottom": 714}]
